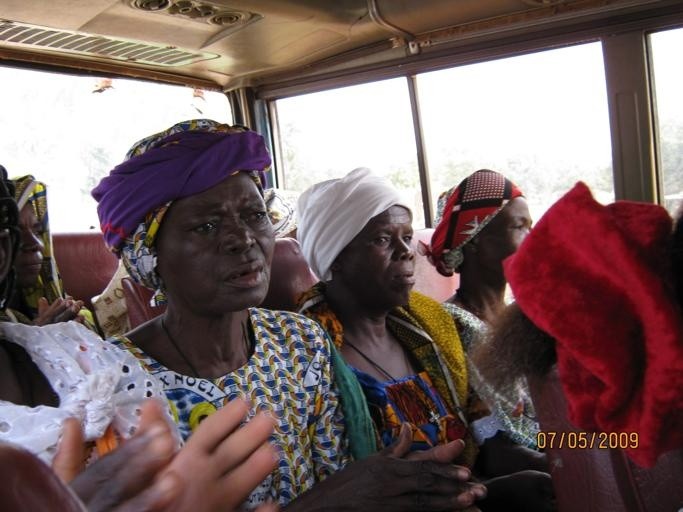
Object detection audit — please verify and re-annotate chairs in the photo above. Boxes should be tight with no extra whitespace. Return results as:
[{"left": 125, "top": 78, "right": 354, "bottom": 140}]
[{"left": 0, "top": 227, "right": 683, "bottom": 512}]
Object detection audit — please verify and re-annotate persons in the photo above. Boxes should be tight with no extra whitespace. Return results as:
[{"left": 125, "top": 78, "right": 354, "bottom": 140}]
[
  {"left": 0, "top": 164, "right": 278, "bottom": 511},
  {"left": 91, "top": 119, "right": 487, "bottom": 511},
  {"left": 263, "top": 166, "right": 556, "bottom": 510},
  {"left": 479, "top": 188, "right": 681, "bottom": 470}
]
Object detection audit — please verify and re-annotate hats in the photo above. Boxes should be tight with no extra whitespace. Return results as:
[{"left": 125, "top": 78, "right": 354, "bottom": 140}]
[
  {"left": 291, "top": 161, "right": 414, "bottom": 288},
  {"left": 362, "top": 199, "right": 381, "bottom": 206},
  {"left": 415, "top": 166, "right": 525, "bottom": 277},
  {"left": 88, "top": 117, "right": 273, "bottom": 256}
]
